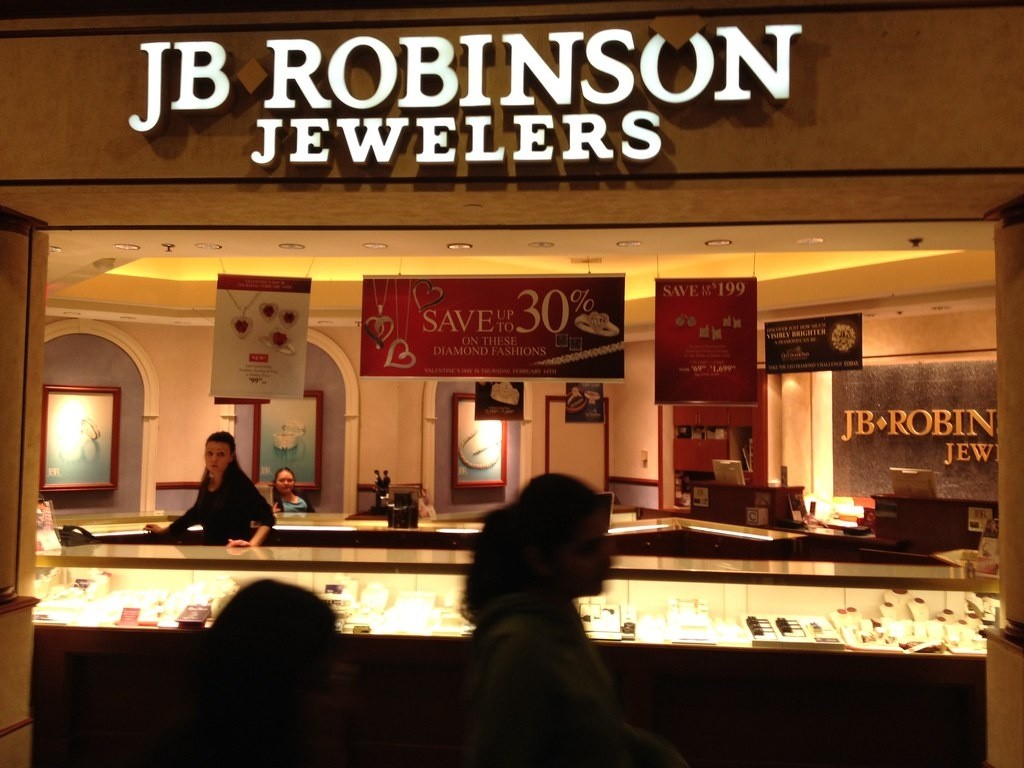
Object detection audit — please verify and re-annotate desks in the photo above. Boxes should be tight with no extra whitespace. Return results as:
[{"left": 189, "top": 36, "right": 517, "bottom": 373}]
[{"left": 686, "top": 479, "right": 999, "bottom": 563}]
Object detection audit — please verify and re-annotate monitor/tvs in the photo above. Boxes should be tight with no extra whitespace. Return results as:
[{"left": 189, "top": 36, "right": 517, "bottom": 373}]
[
  {"left": 890, "top": 467, "right": 938, "bottom": 498},
  {"left": 712, "top": 459, "right": 745, "bottom": 485}
]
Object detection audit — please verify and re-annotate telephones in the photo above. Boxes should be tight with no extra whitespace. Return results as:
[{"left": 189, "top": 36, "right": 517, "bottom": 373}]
[{"left": 54, "top": 524, "right": 94, "bottom": 547}]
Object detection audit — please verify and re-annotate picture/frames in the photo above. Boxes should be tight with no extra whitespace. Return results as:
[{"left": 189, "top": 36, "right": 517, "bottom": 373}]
[
  {"left": 251, "top": 390, "right": 324, "bottom": 492},
  {"left": 39, "top": 384, "right": 121, "bottom": 493},
  {"left": 451, "top": 392, "right": 507, "bottom": 489}
]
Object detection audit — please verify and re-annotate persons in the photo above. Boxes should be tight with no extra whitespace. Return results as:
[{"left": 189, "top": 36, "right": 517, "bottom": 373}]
[
  {"left": 141, "top": 580, "right": 348, "bottom": 768},
  {"left": 143, "top": 431, "right": 277, "bottom": 548},
  {"left": 272, "top": 467, "right": 316, "bottom": 514},
  {"left": 466, "top": 473, "right": 688, "bottom": 768}
]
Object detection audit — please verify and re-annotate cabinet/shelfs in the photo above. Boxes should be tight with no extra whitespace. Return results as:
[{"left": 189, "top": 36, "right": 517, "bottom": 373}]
[{"left": 673, "top": 368, "right": 768, "bottom": 507}]
